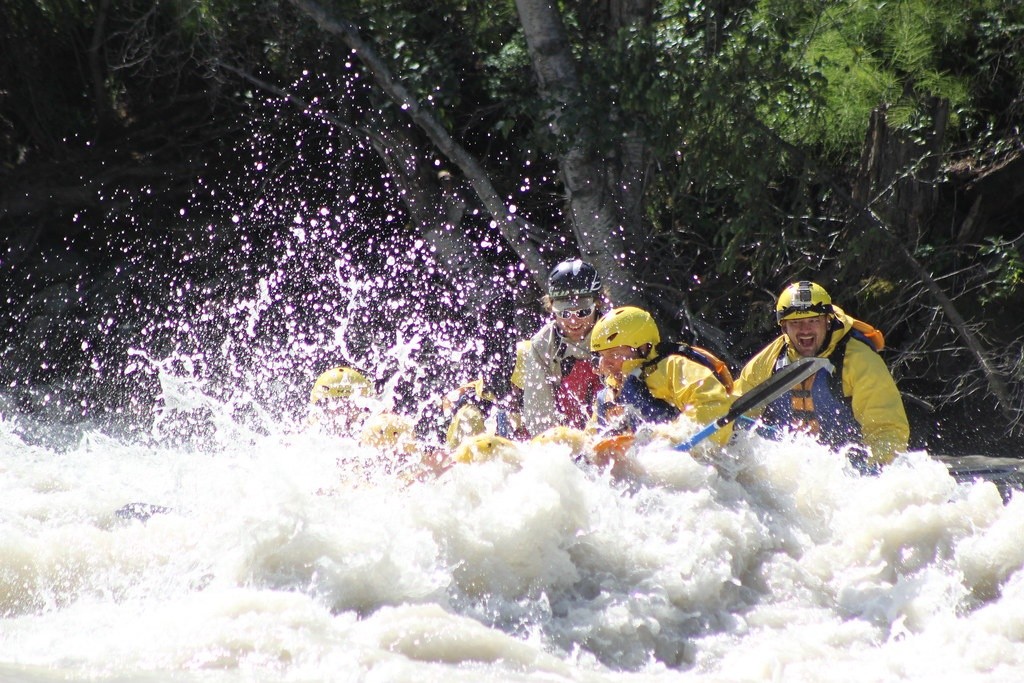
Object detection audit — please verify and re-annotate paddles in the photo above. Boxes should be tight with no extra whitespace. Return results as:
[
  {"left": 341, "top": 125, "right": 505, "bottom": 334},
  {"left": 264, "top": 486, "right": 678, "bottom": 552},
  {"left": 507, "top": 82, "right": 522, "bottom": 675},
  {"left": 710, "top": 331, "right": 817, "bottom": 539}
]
[
  {"left": 675, "top": 356, "right": 830, "bottom": 455},
  {"left": 483, "top": 293, "right": 517, "bottom": 437}
]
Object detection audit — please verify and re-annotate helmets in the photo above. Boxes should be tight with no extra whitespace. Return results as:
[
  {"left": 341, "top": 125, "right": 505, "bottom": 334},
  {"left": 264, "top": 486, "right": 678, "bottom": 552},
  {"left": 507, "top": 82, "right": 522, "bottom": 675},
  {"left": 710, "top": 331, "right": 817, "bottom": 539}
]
[
  {"left": 311, "top": 367, "right": 373, "bottom": 404},
  {"left": 362, "top": 414, "right": 418, "bottom": 455},
  {"left": 532, "top": 427, "right": 588, "bottom": 461},
  {"left": 456, "top": 435, "right": 517, "bottom": 465},
  {"left": 590, "top": 305, "right": 660, "bottom": 352},
  {"left": 547, "top": 256, "right": 605, "bottom": 311},
  {"left": 776, "top": 280, "right": 834, "bottom": 326}
]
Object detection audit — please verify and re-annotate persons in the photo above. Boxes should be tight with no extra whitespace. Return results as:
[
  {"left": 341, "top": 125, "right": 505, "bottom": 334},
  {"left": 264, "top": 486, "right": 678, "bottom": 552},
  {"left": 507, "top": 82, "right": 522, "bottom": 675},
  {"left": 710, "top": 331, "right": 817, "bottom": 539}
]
[
  {"left": 735, "top": 280, "right": 910, "bottom": 477},
  {"left": 312, "top": 256, "right": 733, "bottom": 472}
]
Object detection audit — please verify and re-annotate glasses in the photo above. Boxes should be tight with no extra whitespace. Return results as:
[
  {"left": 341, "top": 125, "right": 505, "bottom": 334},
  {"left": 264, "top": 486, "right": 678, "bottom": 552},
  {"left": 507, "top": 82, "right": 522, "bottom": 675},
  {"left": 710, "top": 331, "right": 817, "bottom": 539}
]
[{"left": 552, "top": 303, "right": 598, "bottom": 319}]
